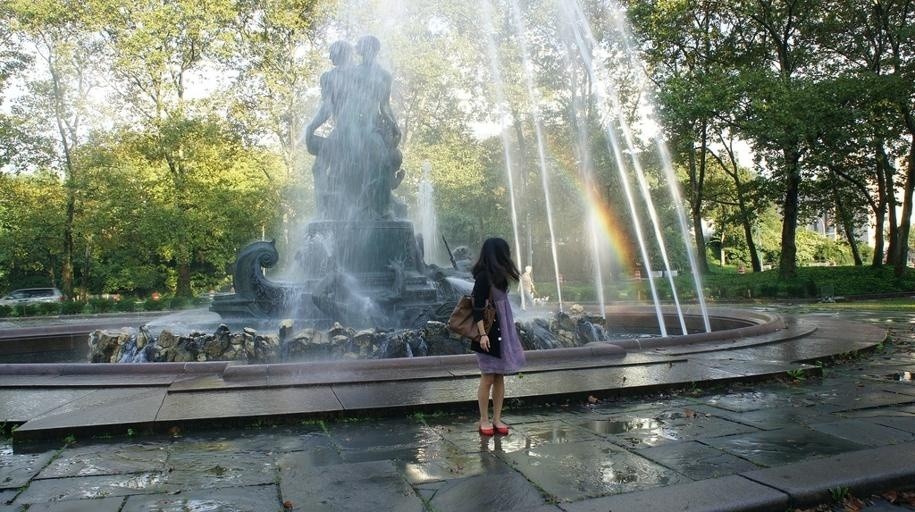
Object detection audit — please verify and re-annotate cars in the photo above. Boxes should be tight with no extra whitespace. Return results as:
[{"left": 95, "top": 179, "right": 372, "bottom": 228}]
[{"left": 0, "top": 287, "right": 68, "bottom": 303}]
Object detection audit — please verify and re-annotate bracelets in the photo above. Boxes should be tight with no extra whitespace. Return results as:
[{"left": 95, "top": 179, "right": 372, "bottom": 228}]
[{"left": 479, "top": 334, "right": 487, "bottom": 337}]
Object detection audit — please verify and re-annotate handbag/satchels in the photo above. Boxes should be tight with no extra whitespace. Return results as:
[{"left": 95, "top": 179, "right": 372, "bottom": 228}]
[
  {"left": 470, "top": 320, "right": 500, "bottom": 358},
  {"left": 449, "top": 295, "right": 495, "bottom": 341}
]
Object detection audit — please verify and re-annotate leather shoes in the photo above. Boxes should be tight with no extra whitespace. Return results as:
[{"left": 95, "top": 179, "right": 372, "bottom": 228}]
[
  {"left": 480, "top": 424, "right": 494, "bottom": 436},
  {"left": 494, "top": 422, "right": 508, "bottom": 435}
]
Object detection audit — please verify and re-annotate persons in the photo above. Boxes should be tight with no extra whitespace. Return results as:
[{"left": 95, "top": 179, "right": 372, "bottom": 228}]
[
  {"left": 516, "top": 264, "right": 539, "bottom": 307},
  {"left": 305, "top": 36, "right": 408, "bottom": 222},
  {"left": 469, "top": 237, "right": 523, "bottom": 437}
]
